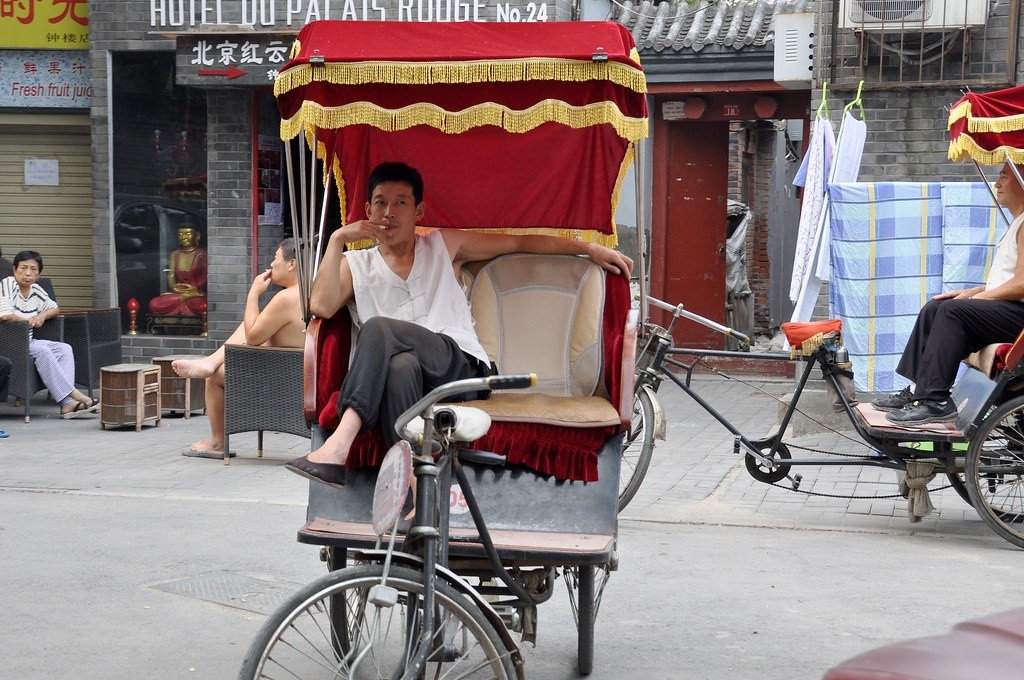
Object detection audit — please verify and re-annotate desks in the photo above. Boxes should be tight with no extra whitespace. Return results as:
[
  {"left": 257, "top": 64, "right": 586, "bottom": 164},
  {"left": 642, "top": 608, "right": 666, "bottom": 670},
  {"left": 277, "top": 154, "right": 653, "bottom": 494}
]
[
  {"left": 98, "top": 364, "right": 161, "bottom": 432},
  {"left": 151, "top": 355, "right": 207, "bottom": 419}
]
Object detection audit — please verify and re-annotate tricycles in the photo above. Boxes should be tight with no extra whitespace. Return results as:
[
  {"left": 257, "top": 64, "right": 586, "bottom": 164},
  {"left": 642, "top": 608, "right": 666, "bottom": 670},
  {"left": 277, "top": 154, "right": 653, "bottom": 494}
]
[
  {"left": 618, "top": 85, "right": 1024, "bottom": 550},
  {"left": 232, "top": 19, "right": 651, "bottom": 680}
]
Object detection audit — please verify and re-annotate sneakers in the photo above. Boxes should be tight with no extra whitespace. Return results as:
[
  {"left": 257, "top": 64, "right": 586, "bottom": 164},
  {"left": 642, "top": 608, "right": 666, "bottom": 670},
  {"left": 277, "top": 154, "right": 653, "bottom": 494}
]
[
  {"left": 886, "top": 397, "right": 959, "bottom": 426},
  {"left": 870, "top": 385, "right": 918, "bottom": 412}
]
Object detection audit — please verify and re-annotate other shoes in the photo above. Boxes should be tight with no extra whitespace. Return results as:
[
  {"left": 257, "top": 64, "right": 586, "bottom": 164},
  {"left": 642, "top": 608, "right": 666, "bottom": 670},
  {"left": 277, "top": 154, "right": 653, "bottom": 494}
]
[
  {"left": 0, "top": 429, "right": 10, "bottom": 438},
  {"left": 284, "top": 453, "right": 347, "bottom": 489},
  {"left": 386, "top": 507, "right": 441, "bottom": 536}
]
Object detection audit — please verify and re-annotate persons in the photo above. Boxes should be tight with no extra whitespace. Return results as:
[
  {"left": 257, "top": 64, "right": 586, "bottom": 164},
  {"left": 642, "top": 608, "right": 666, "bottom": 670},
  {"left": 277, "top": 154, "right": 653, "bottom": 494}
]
[
  {"left": 170, "top": 238, "right": 306, "bottom": 455},
  {"left": 0, "top": 251, "right": 100, "bottom": 420},
  {"left": 286, "top": 161, "right": 634, "bottom": 492},
  {"left": 150, "top": 215, "right": 207, "bottom": 316},
  {"left": 871, "top": 161, "right": 1024, "bottom": 426}
]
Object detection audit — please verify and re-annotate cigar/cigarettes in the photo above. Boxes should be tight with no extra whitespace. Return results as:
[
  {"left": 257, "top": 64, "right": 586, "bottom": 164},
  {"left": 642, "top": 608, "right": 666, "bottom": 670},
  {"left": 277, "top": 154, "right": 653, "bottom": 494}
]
[
  {"left": 378, "top": 225, "right": 388, "bottom": 229},
  {"left": 265, "top": 269, "right": 268, "bottom": 271}
]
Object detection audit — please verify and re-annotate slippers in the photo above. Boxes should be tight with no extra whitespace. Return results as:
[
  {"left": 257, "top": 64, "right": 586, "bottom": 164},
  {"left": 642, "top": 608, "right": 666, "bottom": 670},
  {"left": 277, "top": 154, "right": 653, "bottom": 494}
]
[
  {"left": 182, "top": 445, "right": 237, "bottom": 459},
  {"left": 63, "top": 401, "right": 100, "bottom": 420},
  {"left": 87, "top": 395, "right": 101, "bottom": 414}
]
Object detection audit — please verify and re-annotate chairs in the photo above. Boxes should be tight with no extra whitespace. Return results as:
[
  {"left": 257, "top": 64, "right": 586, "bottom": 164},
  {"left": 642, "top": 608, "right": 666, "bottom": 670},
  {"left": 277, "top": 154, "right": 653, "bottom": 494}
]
[
  {"left": 0, "top": 257, "right": 65, "bottom": 426},
  {"left": 34, "top": 278, "right": 122, "bottom": 414},
  {"left": 224, "top": 343, "right": 312, "bottom": 465}
]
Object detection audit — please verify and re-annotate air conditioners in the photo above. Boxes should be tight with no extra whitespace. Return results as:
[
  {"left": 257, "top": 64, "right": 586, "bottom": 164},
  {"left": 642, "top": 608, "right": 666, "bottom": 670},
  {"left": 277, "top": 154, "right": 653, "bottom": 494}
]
[{"left": 838, "top": 0, "right": 992, "bottom": 35}]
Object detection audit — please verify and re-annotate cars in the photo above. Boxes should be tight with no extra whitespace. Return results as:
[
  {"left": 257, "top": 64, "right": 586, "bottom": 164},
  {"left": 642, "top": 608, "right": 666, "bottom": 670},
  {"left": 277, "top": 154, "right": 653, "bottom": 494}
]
[{"left": 112, "top": 200, "right": 206, "bottom": 304}]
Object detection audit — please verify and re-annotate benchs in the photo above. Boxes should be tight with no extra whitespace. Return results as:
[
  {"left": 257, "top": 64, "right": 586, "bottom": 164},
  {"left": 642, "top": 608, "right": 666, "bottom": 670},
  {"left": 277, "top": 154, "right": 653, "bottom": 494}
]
[
  {"left": 302, "top": 252, "right": 640, "bottom": 485},
  {"left": 961, "top": 329, "right": 1024, "bottom": 379}
]
